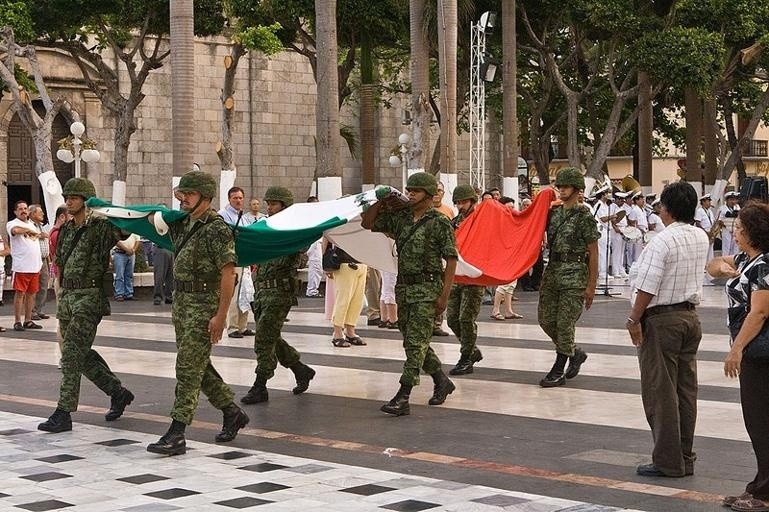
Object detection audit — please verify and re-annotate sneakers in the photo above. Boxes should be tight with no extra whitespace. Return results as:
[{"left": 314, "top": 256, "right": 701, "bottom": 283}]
[
  {"left": 367, "top": 316, "right": 450, "bottom": 337},
  {"left": 38, "top": 409, "right": 73, "bottom": 432},
  {"left": 450, "top": 358, "right": 473, "bottom": 374},
  {"left": 11, "top": 310, "right": 50, "bottom": 332},
  {"left": 105, "top": 388, "right": 135, "bottom": 420},
  {"left": 113, "top": 294, "right": 173, "bottom": 305},
  {"left": 566, "top": 350, "right": 587, "bottom": 379},
  {"left": 486, "top": 285, "right": 542, "bottom": 305},
  {"left": 471, "top": 350, "right": 483, "bottom": 364},
  {"left": 598, "top": 271, "right": 631, "bottom": 282},
  {"left": 722, "top": 491, "right": 767, "bottom": 511}
]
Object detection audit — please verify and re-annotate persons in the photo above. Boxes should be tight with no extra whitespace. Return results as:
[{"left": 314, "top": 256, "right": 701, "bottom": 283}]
[
  {"left": 624, "top": 183, "right": 709, "bottom": 476},
  {"left": 706, "top": 202, "right": 769, "bottom": 511},
  {"left": 146, "top": 170, "right": 249, "bottom": 456},
  {"left": 6, "top": 187, "right": 266, "bottom": 338},
  {"left": 360, "top": 171, "right": 457, "bottom": 416},
  {"left": 38, "top": 177, "right": 134, "bottom": 433},
  {"left": 447, "top": 184, "right": 483, "bottom": 374},
  {"left": 536, "top": 168, "right": 601, "bottom": 388},
  {"left": 306, "top": 189, "right": 741, "bottom": 347},
  {"left": 241, "top": 187, "right": 316, "bottom": 405}
]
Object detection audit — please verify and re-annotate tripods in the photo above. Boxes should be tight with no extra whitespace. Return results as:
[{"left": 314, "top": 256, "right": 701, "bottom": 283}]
[{"left": 595, "top": 204, "right": 621, "bottom": 297}]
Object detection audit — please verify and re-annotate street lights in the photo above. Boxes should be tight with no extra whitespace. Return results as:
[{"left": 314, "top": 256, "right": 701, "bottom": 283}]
[
  {"left": 469, "top": 11, "right": 499, "bottom": 190},
  {"left": 56, "top": 119, "right": 102, "bottom": 181},
  {"left": 390, "top": 134, "right": 414, "bottom": 194}
]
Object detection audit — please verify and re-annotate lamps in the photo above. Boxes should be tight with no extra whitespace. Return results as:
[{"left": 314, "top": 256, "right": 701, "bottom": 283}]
[
  {"left": 480, "top": 61, "right": 499, "bottom": 83},
  {"left": 478, "top": 9, "right": 498, "bottom": 34}
]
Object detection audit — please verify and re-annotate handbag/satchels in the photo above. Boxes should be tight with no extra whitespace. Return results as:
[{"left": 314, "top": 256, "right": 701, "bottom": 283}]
[{"left": 730, "top": 304, "right": 766, "bottom": 351}]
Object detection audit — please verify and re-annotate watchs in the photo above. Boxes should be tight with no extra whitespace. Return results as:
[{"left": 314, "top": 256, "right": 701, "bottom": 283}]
[{"left": 626, "top": 317, "right": 639, "bottom": 325}]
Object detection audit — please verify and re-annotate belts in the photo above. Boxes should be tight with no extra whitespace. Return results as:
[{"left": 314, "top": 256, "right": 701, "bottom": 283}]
[{"left": 644, "top": 303, "right": 696, "bottom": 315}]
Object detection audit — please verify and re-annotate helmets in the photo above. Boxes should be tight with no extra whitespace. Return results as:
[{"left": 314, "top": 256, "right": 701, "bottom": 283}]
[
  {"left": 61, "top": 177, "right": 96, "bottom": 202},
  {"left": 452, "top": 184, "right": 479, "bottom": 204},
  {"left": 262, "top": 186, "right": 295, "bottom": 207},
  {"left": 178, "top": 170, "right": 217, "bottom": 196},
  {"left": 555, "top": 167, "right": 586, "bottom": 188},
  {"left": 405, "top": 172, "right": 440, "bottom": 197}
]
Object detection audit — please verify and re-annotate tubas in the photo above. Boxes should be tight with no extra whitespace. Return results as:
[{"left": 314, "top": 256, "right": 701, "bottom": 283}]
[
  {"left": 709, "top": 219, "right": 726, "bottom": 237},
  {"left": 610, "top": 175, "right": 643, "bottom": 198}
]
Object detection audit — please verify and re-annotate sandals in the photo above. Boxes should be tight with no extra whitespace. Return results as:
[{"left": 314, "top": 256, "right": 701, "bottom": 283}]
[
  {"left": 345, "top": 335, "right": 367, "bottom": 345},
  {"left": 332, "top": 337, "right": 351, "bottom": 347},
  {"left": 490, "top": 313, "right": 523, "bottom": 321}
]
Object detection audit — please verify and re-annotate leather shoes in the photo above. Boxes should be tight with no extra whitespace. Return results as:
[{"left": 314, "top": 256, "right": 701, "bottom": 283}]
[
  {"left": 228, "top": 329, "right": 255, "bottom": 338},
  {"left": 636, "top": 457, "right": 696, "bottom": 477}
]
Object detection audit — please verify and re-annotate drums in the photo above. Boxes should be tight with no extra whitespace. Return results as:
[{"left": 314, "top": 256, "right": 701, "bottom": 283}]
[
  {"left": 622, "top": 226, "right": 643, "bottom": 244},
  {"left": 644, "top": 230, "right": 659, "bottom": 246}
]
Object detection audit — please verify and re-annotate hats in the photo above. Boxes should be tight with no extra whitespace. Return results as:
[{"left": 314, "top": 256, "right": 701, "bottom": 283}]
[{"left": 593, "top": 186, "right": 741, "bottom": 202}]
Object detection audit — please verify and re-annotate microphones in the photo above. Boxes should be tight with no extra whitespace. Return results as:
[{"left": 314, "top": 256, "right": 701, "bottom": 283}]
[{"left": 606, "top": 198, "right": 615, "bottom": 206}]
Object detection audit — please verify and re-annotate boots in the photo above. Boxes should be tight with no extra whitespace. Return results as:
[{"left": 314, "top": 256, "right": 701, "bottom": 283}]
[
  {"left": 539, "top": 352, "right": 569, "bottom": 387},
  {"left": 381, "top": 382, "right": 413, "bottom": 415},
  {"left": 147, "top": 418, "right": 186, "bottom": 455},
  {"left": 241, "top": 380, "right": 269, "bottom": 404},
  {"left": 290, "top": 360, "right": 315, "bottom": 395},
  {"left": 215, "top": 403, "right": 250, "bottom": 442},
  {"left": 429, "top": 371, "right": 456, "bottom": 406}
]
